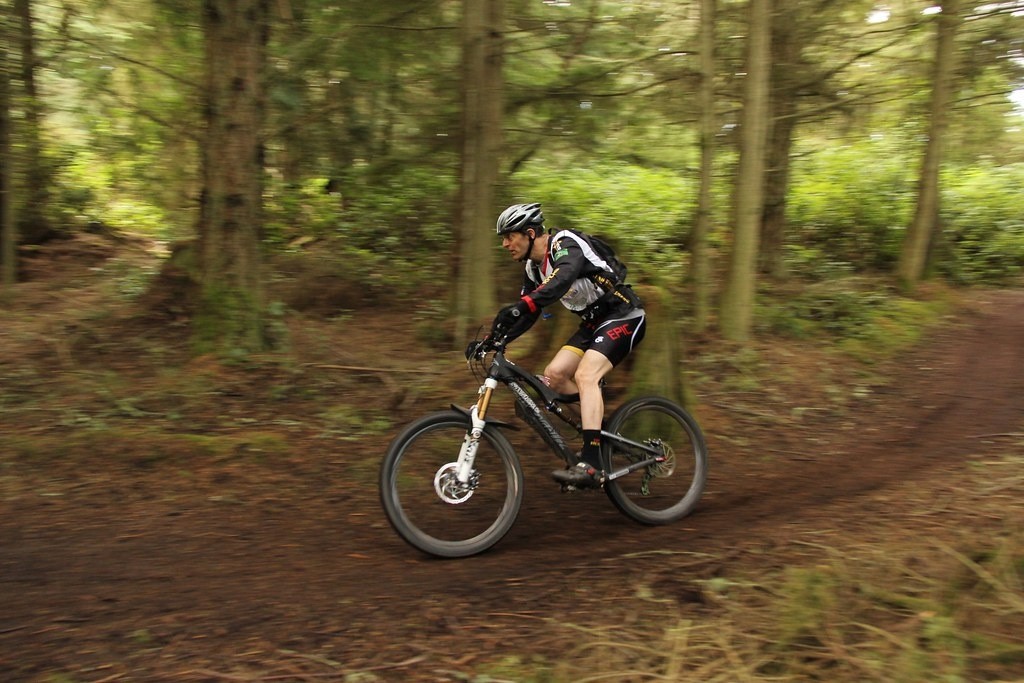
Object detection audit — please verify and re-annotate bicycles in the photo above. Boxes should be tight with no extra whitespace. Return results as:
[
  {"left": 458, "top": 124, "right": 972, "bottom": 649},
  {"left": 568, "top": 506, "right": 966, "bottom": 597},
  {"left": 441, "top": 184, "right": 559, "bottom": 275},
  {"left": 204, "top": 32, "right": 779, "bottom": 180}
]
[{"left": 379, "top": 308, "right": 709, "bottom": 560}]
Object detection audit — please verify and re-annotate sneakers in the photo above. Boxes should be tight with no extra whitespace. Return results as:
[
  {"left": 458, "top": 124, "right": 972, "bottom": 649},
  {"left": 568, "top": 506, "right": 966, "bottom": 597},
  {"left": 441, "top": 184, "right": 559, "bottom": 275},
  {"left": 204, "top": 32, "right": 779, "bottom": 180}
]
[
  {"left": 552, "top": 460, "right": 605, "bottom": 489},
  {"left": 602, "top": 419, "right": 608, "bottom": 430}
]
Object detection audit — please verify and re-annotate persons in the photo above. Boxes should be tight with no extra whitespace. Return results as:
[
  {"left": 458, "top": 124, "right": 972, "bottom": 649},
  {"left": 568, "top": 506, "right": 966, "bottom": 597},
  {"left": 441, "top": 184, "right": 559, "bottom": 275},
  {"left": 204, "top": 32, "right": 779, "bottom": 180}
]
[{"left": 465, "top": 201, "right": 648, "bottom": 489}]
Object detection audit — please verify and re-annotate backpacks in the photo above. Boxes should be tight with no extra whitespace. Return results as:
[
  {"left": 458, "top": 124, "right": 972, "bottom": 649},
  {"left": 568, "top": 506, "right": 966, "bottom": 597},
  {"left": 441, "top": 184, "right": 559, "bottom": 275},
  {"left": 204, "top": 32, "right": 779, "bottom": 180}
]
[{"left": 531, "top": 227, "right": 627, "bottom": 284}]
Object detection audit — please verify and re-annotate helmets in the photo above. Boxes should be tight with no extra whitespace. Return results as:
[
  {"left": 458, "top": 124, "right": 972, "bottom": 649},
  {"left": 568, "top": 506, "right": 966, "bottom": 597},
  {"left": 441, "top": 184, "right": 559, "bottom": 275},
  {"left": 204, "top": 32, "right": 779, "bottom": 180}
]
[{"left": 497, "top": 203, "right": 545, "bottom": 235}]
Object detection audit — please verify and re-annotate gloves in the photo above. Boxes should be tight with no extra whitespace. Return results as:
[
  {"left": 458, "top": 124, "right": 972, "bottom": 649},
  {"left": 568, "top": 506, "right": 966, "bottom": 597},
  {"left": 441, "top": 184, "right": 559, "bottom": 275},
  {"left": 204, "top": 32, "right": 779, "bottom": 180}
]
[
  {"left": 466, "top": 342, "right": 491, "bottom": 361},
  {"left": 498, "top": 300, "right": 529, "bottom": 328}
]
